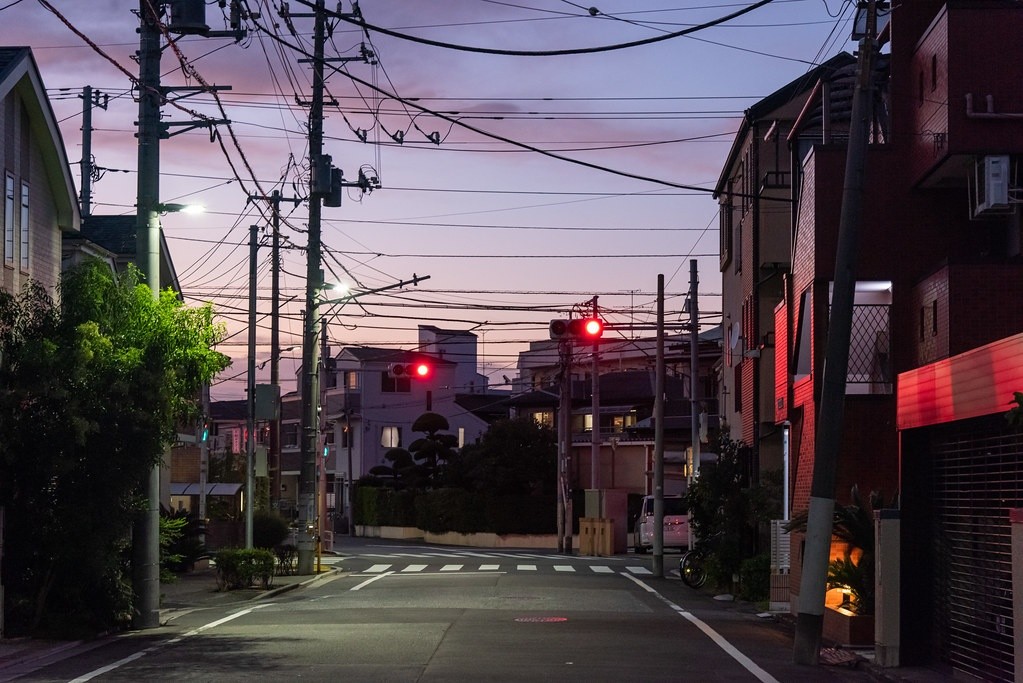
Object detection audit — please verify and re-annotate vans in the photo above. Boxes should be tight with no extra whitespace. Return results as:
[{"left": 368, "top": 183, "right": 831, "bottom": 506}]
[{"left": 631, "top": 494, "right": 688, "bottom": 555}]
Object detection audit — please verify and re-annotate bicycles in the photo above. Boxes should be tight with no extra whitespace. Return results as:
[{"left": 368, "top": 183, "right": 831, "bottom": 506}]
[
  {"left": 679, "top": 528, "right": 725, "bottom": 588},
  {"left": 276, "top": 550, "right": 299, "bottom": 576}
]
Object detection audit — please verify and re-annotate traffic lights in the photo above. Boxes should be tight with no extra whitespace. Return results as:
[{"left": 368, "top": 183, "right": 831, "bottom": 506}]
[
  {"left": 198, "top": 416, "right": 210, "bottom": 443},
  {"left": 387, "top": 362, "right": 431, "bottom": 379},
  {"left": 550, "top": 318, "right": 602, "bottom": 340},
  {"left": 322, "top": 443, "right": 329, "bottom": 458}
]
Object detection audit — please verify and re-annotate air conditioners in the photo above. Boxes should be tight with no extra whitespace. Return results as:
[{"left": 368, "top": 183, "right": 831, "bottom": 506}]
[{"left": 973, "top": 155, "right": 1011, "bottom": 217}]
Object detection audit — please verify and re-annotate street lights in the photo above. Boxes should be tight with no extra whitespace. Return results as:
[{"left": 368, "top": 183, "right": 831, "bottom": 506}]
[
  {"left": 123, "top": 200, "right": 204, "bottom": 630},
  {"left": 293, "top": 279, "right": 352, "bottom": 576}
]
